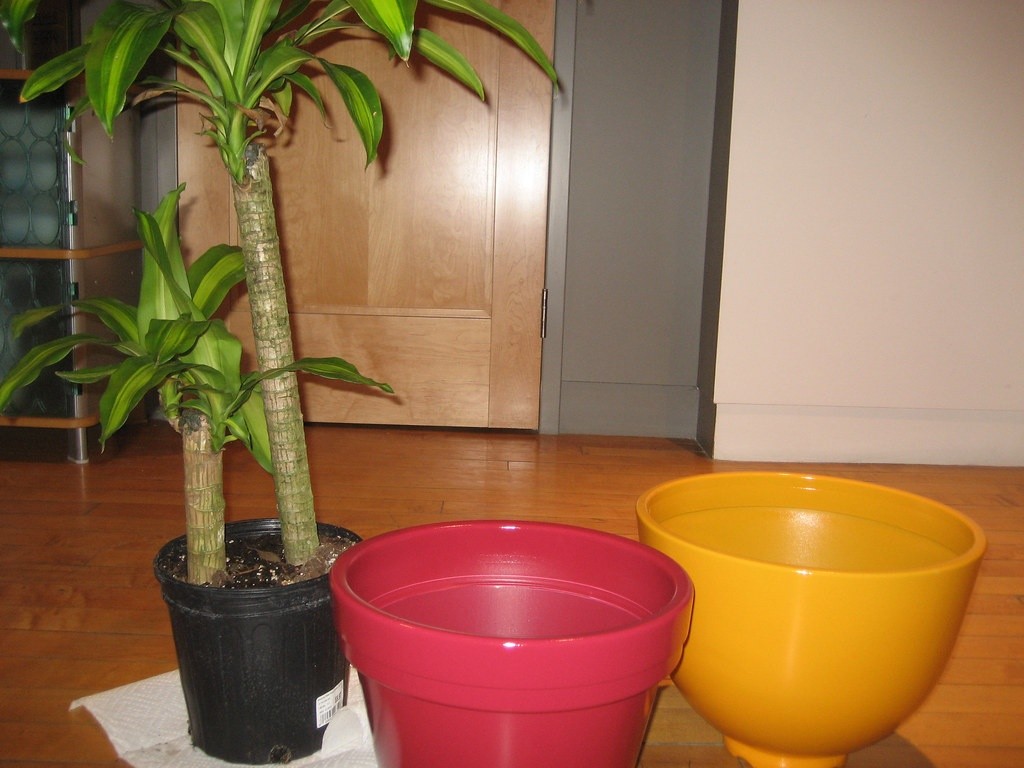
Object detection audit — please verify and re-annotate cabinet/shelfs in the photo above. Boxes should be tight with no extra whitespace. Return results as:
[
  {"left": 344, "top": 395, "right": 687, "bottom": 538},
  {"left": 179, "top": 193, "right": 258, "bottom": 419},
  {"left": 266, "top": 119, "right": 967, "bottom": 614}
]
[{"left": 0, "top": 0, "right": 141, "bottom": 465}]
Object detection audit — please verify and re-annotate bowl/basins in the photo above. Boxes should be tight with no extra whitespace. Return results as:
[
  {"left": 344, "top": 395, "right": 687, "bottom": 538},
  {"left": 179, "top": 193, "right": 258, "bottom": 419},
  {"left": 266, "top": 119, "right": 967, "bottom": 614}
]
[{"left": 636, "top": 470, "right": 986, "bottom": 768}]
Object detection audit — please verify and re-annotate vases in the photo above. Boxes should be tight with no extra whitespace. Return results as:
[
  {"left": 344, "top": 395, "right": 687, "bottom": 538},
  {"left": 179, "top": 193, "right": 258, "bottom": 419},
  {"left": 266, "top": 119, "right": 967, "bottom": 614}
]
[
  {"left": 634, "top": 470, "right": 989, "bottom": 768},
  {"left": 329, "top": 519, "right": 696, "bottom": 768}
]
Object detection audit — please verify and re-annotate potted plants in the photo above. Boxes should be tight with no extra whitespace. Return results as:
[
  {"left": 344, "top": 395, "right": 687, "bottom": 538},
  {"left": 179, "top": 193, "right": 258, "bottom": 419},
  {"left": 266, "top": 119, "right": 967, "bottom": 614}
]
[{"left": 0, "top": 0, "right": 561, "bottom": 766}]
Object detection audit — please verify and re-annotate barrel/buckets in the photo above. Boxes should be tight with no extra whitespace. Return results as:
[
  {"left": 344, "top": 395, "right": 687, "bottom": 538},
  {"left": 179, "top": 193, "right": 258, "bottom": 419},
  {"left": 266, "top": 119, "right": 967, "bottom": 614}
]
[{"left": 330, "top": 520, "right": 694, "bottom": 768}]
